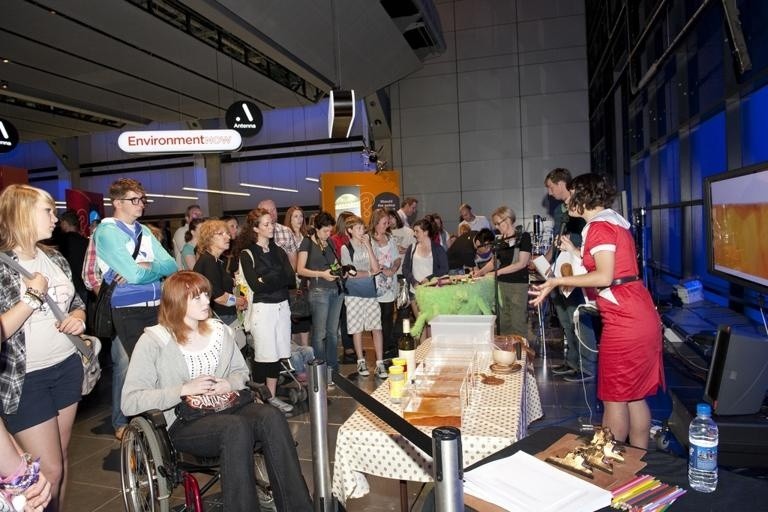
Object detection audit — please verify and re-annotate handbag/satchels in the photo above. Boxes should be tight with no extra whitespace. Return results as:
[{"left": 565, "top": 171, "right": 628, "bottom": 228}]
[
  {"left": 290, "top": 236, "right": 312, "bottom": 321},
  {"left": 395, "top": 242, "right": 416, "bottom": 310},
  {"left": 93, "top": 231, "right": 143, "bottom": 338},
  {"left": 0, "top": 251, "right": 102, "bottom": 396},
  {"left": 174, "top": 388, "right": 253, "bottom": 425}
]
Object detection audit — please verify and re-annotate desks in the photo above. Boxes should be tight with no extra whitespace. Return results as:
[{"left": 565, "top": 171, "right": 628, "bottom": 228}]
[
  {"left": 528, "top": 254, "right": 554, "bottom": 358},
  {"left": 415, "top": 276, "right": 496, "bottom": 338},
  {"left": 338, "top": 336, "right": 527, "bottom": 512},
  {"left": 429, "top": 425, "right": 767, "bottom": 511}
]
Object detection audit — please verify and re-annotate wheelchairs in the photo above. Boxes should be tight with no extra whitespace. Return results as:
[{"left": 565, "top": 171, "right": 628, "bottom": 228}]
[{"left": 108, "top": 305, "right": 298, "bottom": 512}]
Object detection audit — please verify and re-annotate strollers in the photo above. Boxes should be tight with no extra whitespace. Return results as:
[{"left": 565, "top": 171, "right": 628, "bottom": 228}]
[{"left": 280, "top": 357, "right": 309, "bottom": 404}]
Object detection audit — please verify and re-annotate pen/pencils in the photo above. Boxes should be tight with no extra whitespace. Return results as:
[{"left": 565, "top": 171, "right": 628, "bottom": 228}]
[{"left": 611, "top": 474, "right": 688, "bottom": 512}]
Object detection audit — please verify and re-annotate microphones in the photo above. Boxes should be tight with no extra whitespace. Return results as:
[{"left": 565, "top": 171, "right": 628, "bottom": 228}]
[
  {"left": 531, "top": 214, "right": 541, "bottom": 245},
  {"left": 554, "top": 214, "right": 571, "bottom": 248}
]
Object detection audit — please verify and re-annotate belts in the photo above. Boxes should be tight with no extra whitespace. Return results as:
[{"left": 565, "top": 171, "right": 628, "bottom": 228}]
[{"left": 597, "top": 274, "right": 641, "bottom": 292}]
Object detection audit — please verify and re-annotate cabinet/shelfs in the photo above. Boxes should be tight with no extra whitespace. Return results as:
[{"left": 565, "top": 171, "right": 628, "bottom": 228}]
[{"left": 320, "top": 170, "right": 400, "bottom": 233}]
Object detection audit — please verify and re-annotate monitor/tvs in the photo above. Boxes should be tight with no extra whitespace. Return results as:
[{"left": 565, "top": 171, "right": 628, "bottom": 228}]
[{"left": 701, "top": 159, "right": 768, "bottom": 295}]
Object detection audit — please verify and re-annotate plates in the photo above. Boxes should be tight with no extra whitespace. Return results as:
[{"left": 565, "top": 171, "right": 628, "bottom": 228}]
[{"left": 489, "top": 364, "right": 523, "bottom": 374}]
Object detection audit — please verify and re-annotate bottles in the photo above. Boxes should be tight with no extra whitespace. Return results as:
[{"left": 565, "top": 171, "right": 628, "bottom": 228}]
[
  {"left": 397, "top": 319, "right": 416, "bottom": 386},
  {"left": 392, "top": 358, "right": 408, "bottom": 382},
  {"left": 388, "top": 365, "right": 404, "bottom": 405},
  {"left": 687, "top": 403, "right": 718, "bottom": 493}
]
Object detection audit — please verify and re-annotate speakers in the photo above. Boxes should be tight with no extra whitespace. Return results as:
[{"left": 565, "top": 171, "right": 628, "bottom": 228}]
[{"left": 701, "top": 323, "right": 768, "bottom": 416}]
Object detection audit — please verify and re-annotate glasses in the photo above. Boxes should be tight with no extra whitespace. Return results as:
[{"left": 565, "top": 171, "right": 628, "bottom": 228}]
[
  {"left": 497, "top": 219, "right": 506, "bottom": 226},
  {"left": 214, "top": 231, "right": 231, "bottom": 237},
  {"left": 120, "top": 196, "right": 148, "bottom": 205}
]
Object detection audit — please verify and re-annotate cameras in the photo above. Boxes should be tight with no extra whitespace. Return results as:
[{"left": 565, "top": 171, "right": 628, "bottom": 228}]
[{"left": 329, "top": 264, "right": 357, "bottom": 279}]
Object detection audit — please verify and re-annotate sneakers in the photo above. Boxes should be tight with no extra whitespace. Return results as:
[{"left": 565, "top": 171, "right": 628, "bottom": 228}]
[
  {"left": 551, "top": 363, "right": 575, "bottom": 374},
  {"left": 269, "top": 396, "right": 294, "bottom": 414},
  {"left": 114, "top": 424, "right": 129, "bottom": 439},
  {"left": 373, "top": 361, "right": 387, "bottom": 378},
  {"left": 355, "top": 359, "right": 370, "bottom": 376},
  {"left": 563, "top": 369, "right": 594, "bottom": 382}
]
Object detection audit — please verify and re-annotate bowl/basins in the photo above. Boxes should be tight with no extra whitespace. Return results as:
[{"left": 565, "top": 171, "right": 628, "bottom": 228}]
[{"left": 494, "top": 350, "right": 517, "bottom": 367}]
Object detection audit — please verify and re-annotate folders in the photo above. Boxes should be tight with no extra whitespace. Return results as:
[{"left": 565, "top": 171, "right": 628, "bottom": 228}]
[
  {"left": 464, "top": 450, "right": 619, "bottom": 512},
  {"left": 544, "top": 429, "right": 648, "bottom": 474},
  {"left": 545, "top": 425, "right": 647, "bottom": 460},
  {"left": 533, "top": 440, "right": 638, "bottom": 490}
]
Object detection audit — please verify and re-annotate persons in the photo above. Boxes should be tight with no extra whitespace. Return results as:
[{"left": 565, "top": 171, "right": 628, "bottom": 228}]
[
  {"left": 120, "top": 271, "right": 316, "bottom": 512},
  {"left": 181, "top": 218, "right": 204, "bottom": 271},
  {"left": 93, "top": 178, "right": 180, "bottom": 363},
  {"left": 171, "top": 203, "right": 203, "bottom": 265},
  {"left": 396, "top": 197, "right": 419, "bottom": 228},
  {"left": 529, "top": 168, "right": 599, "bottom": 381},
  {"left": 258, "top": 198, "right": 298, "bottom": 271},
  {"left": 527, "top": 173, "right": 667, "bottom": 450},
  {"left": 469, "top": 207, "right": 532, "bottom": 342},
  {"left": 53, "top": 209, "right": 90, "bottom": 301},
  {"left": 458, "top": 203, "right": 491, "bottom": 233},
  {"left": 296, "top": 211, "right": 345, "bottom": 382},
  {"left": 446, "top": 227, "right": 496, "bottom": 275},
  {"left": 402, "top": 219, "right": 449, "bottom": 343},
  {"left": 230, "top": 207, "right": 297, "bottom": 415},
  {"left": 339, "top": 216, "right": 391, "bottom": 379},
  {"left": 435, "top": 214, "right": 451, "bottom": 251},
  {"left": 330, "top": 210, "right": 355, "bottom": 262},
  {"left": 363, "top": 209, "right": 402, "bottom": 367},
  {"left": 0, "top": 411, "right": 52, "bottom": 507},
  {"left": 191, "top": 220, "right": 248, "bottom": 351},
  {"left": 0, "top": 183, "right": 86, "bottom": 507},
  {"left": 284, "top": 206, "right": 309, "bottom": 251},
  {"left": 224, "top": 218, "right": 239, "bottom": 241}
]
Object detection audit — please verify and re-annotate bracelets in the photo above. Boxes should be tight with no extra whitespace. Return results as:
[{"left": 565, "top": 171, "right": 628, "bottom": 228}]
[
  {"left": 0, "top": 459, "right": 42, "bottom": 497},
  {"left": 22, "top": 286, "right": 49, "bottom": 311}
]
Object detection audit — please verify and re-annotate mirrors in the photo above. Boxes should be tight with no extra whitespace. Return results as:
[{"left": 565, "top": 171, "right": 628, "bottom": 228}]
[{"left": 335, "top": 185, "right": 361, "bottom": 223}]
